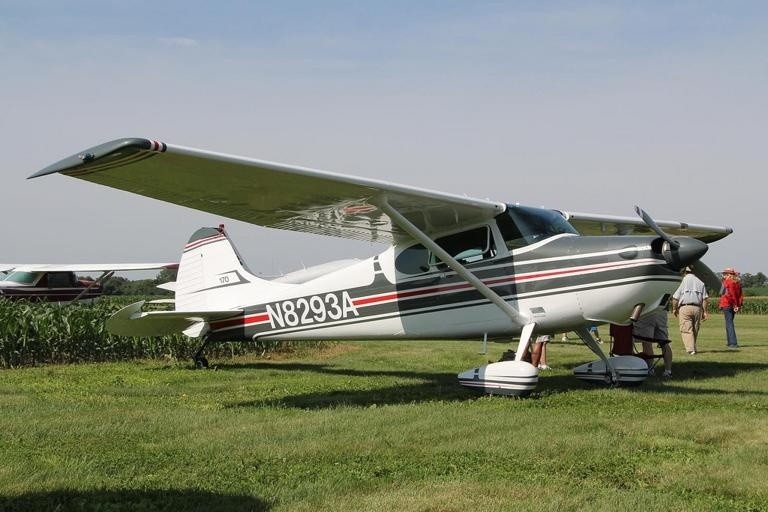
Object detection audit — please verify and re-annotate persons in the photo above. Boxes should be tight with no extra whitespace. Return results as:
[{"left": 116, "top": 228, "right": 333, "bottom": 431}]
[
  {"left": 631, "top": 299, "right": 674, "bottom": 378},
  {"left": 718, "top": 266, "right": 744, "bottom": 352},
  {"left": 672, "top": 265, "right": 709, "bottom": 356},
  {"left": 591, "top": 325, "right": 603, "bottom": 345},
  {"left": 531, "top": 334, "right": 553, "bottom": 371},
  {"left": 562, "top": 333, "right": 569, "bottom": 344}
]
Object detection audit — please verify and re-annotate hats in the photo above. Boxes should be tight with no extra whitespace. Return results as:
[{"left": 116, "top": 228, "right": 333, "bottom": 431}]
[{"left": 722, "top": 268, "right": 734, "bottom": 273}]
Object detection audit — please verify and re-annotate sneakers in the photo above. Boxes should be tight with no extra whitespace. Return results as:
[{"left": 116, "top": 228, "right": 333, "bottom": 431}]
[
  {"left": 538, "top": 363, "right": 552, "bottom": 371},
  {"left": 662, "top": 371, "right": 673, "bottom": 379},
  {"left": 563, "top": 336, "right": 568, "bottom": 341},
  {"left": 690, "top": 350, "right": 696, "bottom": 355},
  {"left": 728, "top": 344, "right": 738, "bottom": 348},
  {"left": 596, "top": 337, "right": 603, "bottom": 344}
]
